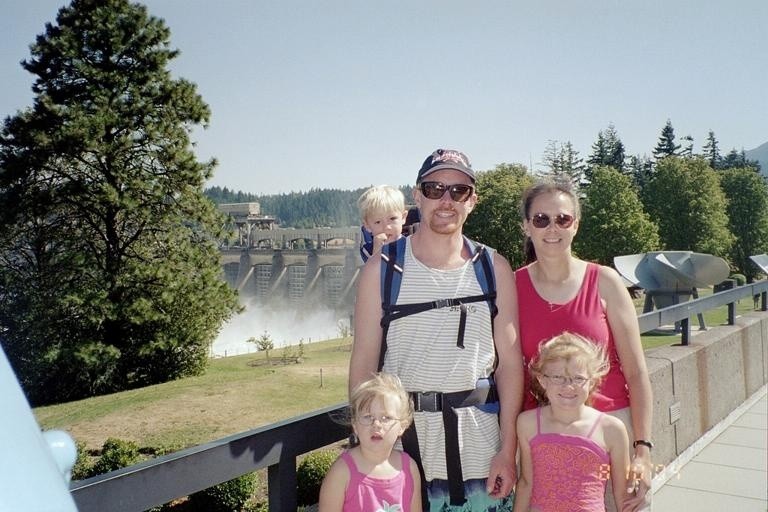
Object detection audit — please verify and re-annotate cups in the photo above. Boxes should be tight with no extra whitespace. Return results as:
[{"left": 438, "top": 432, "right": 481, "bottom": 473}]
[
  {"left": 355, "top": 412, "right": 402, "bottom": 425},
  {"left": 543, "top": 373, "right": 591, "bottom": 386},
  {"left": 418, "top": 181, "right": 475, "bottom": 204},
  {"left": 528, "top": 212, "right": 576, "bottom": 229}
]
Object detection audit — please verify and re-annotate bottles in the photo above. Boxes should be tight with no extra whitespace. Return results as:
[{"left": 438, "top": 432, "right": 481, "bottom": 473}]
[{"left": 416, "top": 148, "right": 477, "bottom": 183}]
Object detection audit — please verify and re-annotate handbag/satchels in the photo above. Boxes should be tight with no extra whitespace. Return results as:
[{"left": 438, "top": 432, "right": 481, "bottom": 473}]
[{"left": 632, "top": 438, "right": 655, "bottom": 452}]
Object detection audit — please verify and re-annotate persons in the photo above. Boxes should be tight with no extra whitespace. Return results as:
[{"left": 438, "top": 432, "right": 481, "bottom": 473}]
[
  {"left": 503, "top": 185, "right": 656, "bottom": 511},
  {"left": 349, "top": 146, "right": 523, "bottom": 511},
  {"left": 317, "top": 370, "right": 427, "bottom": 512},
  {"left": 357, "top": 182, "right": 425, "bottom": 265},
  {"left": 510, "top": 330, "right": 637, "bottom": 511}
]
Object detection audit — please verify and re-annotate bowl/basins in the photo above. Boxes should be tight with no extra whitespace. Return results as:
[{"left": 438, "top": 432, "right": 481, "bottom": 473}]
[{"left": 538, "top": 281, "right": 566, "bottom": 312}]
